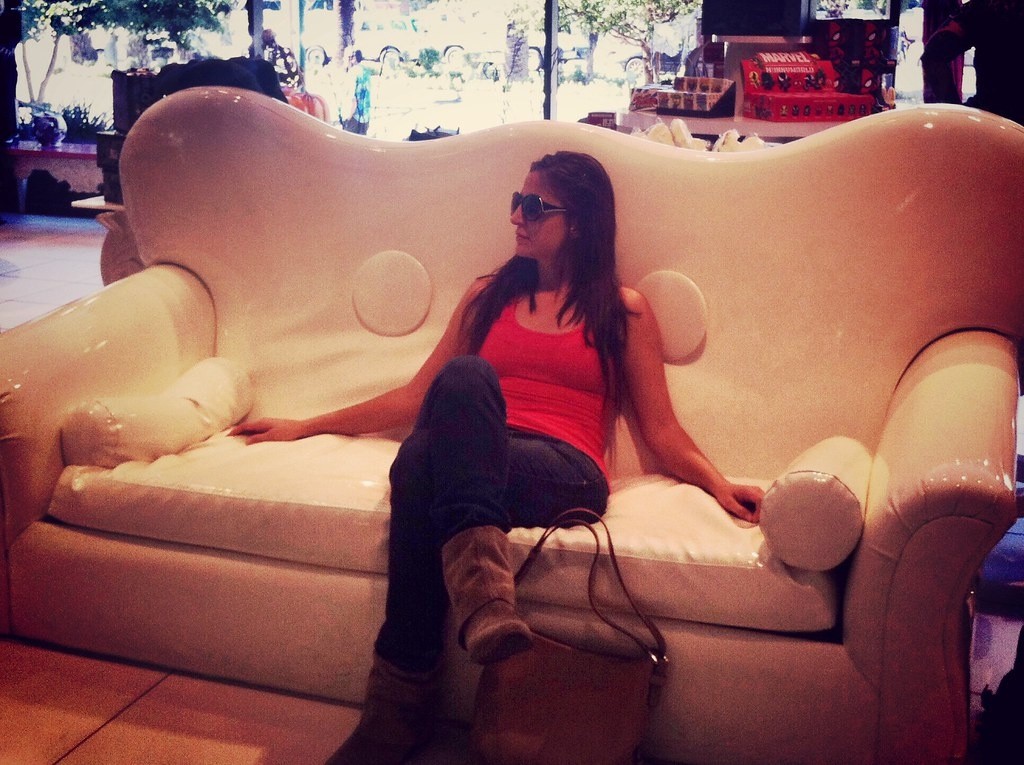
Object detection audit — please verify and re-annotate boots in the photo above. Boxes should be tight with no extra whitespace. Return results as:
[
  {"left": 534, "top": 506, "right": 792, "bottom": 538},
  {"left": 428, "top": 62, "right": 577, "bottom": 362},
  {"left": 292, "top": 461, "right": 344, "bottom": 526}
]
[
  {"left": 441, "top": 525, "right": 531, "bottom": 663},
  {"left": 326, "top": 650, "right": 441, "bottom": 765}
]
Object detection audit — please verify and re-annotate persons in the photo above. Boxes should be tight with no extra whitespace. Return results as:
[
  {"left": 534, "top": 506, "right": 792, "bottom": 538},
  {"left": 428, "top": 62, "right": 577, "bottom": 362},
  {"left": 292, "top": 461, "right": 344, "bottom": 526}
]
[
  {"left": 226, "top": 150, "right": 765, "bottom": 765},
  {"left": 334, "top": 46, "right": 371, "bottom": 136},
  {"left": 921, "top": 0, "right": 1024, "bottom": 126}
]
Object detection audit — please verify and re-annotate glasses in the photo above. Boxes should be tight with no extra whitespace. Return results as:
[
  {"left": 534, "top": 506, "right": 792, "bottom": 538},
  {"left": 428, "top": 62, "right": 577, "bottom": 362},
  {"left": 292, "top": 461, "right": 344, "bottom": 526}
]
[{"left": 511, "top": 191, "right": 570, "bottom": 222}]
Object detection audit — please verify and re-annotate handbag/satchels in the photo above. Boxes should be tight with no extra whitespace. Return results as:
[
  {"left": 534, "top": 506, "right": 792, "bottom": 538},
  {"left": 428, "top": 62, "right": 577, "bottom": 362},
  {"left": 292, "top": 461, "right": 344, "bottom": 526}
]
[{"left": 459, "top": 507, "right": 668, "bottom": 765}]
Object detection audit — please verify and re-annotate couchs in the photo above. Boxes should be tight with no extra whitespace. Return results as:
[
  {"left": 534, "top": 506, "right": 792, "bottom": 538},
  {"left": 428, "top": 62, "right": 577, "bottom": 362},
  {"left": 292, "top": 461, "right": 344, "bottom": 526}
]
[{"left": 0, "top": 87, "right": 1024, "bottom": 765}]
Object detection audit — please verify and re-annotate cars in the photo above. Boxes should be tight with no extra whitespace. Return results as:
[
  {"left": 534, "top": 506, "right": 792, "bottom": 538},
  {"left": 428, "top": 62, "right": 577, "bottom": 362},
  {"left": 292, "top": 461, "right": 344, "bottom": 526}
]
[{"left": 181, "top": 0, "right": 703, "bottom": 89}]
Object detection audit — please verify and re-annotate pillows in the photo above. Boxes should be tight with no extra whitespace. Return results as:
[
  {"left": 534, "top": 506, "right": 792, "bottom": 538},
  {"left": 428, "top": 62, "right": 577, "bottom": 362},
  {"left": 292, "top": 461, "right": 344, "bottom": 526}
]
[
  {"left": 759, "top": 437, "right": 872, "bottom": 572},
  {"left": 62, "top": 356, "right": 256, "bottom": 470}
]
